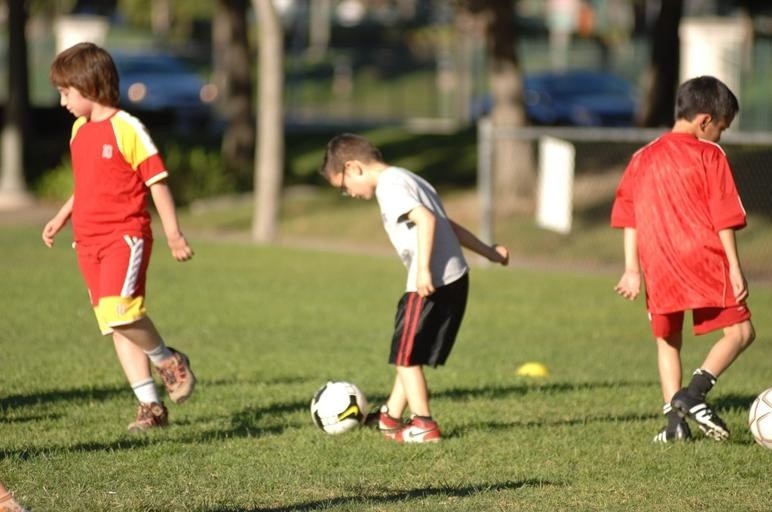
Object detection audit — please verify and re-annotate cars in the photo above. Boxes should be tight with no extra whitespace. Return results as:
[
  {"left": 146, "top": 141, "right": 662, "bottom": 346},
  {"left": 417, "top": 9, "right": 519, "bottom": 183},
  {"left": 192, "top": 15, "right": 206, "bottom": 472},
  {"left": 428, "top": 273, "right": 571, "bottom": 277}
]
[
  {"left": 111, "top": 51, "right": 211, "bottom": 126},
  {"left": 469, "top": 67, "right": 633, "bottom": 130}
]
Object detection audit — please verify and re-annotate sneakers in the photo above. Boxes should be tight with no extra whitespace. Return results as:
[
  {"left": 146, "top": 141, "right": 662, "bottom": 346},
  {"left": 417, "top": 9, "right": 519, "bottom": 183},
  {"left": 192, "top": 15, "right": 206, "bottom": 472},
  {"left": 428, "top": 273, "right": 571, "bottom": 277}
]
[
  {"left": 127, "top": 402, "right": 168, "bottom": 430},
  {"left": 652, "top": 423, "right": 691, "bottom": 443},
  {"left": 367, "top": 406, "right": 402, "bottom": 434},
  {"left": 155, "top": 347, "right": 194, "bottom": 405},
  {"left": 671, "top": 388, "right": 730, "bottom": 441},
  {"left": 385, "top": 414, "right": 441, "bottom": 443}
]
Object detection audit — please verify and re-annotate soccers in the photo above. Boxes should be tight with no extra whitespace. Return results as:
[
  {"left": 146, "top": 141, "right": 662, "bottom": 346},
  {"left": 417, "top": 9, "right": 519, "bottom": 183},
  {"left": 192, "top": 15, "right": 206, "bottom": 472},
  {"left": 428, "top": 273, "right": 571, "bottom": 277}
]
[
  {"left": 749, "top": 387, "right": 772, "bottom": 452},
  {"left": 310, "top": 380, "right": 368, "bottom": 437}
]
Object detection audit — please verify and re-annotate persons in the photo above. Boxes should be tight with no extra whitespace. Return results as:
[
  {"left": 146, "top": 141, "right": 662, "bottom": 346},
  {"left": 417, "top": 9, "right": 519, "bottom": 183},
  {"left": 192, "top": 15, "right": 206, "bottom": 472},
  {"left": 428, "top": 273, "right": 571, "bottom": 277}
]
[
  {"left": 41, "top": 41, "right": 198, "bottom": 429},
  {"left": 609, "top": 73, "right": 757, "bottom": 452},
  {"left": 313, "top": 130, "right": 510, "bottom": 443}
]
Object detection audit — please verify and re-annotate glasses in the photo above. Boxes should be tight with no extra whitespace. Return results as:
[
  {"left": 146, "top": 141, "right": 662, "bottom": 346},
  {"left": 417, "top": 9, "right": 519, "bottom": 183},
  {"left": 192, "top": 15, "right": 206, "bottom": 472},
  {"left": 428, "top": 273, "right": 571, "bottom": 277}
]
[{"left": 339, "top": 166, "right": 349, "bottom": 197}]
